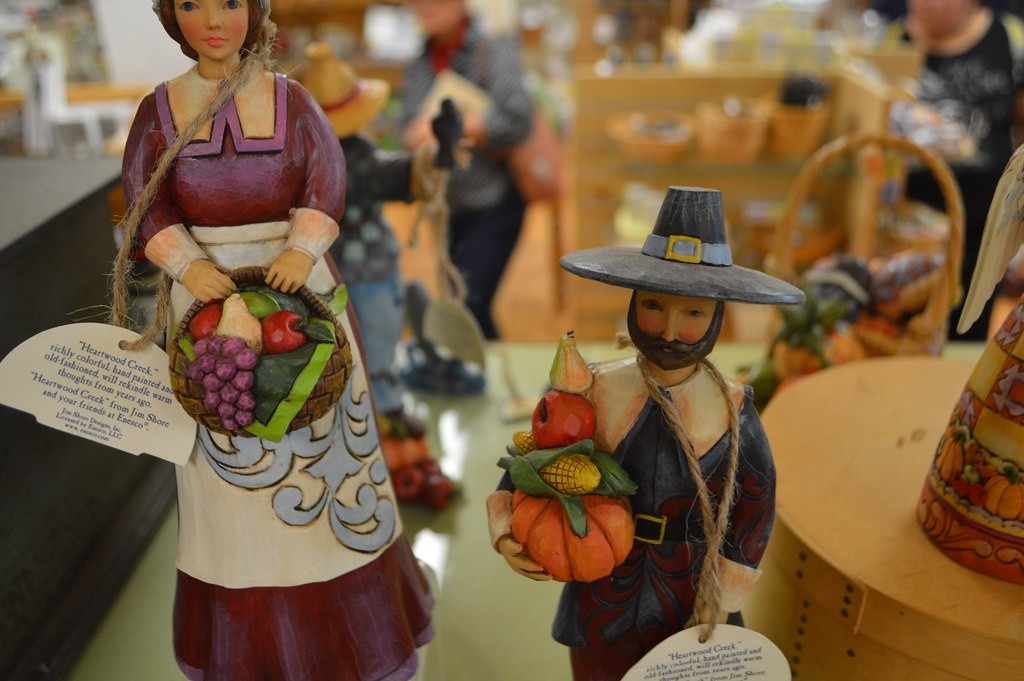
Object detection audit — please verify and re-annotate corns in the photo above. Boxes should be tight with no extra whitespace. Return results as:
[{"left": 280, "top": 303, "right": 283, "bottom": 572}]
[{"left": 513, "top": 430, "right": 601, "bottom": 496}]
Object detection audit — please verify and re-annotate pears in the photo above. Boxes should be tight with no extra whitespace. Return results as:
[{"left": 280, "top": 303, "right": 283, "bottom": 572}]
[
  {"left": 213, "top": 293, "right": 262, "bottom": 360},
  {"left": 549, "top": 331, "right": 593, "bottom": 394}
]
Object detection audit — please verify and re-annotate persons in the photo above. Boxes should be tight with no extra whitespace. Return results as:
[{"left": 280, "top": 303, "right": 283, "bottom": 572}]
[
  {"left": 281, "top": 40, "right": 475, "bottom": 443},
  {"left": 396, "top": 1, "right": 568, "bottom": 342},
  {"left": 486, "top": 187, "right": 809, "bottom": 681},
  {"left": 123, "top": 1, "right": 435, "bottom": 681},
  {"left": 820, "top": 0, "right": 1016, "bottom": 339}
]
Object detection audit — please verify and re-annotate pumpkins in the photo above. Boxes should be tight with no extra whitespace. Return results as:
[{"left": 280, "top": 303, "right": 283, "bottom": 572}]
[{"left": 510, "top": 487, "right": 634, "bottom": 583}]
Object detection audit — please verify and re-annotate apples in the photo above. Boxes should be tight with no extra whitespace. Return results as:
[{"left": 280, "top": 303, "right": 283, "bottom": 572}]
[
  {"left": 189, "top": 303, "right": 223, "bottom": 341},
  {"left": 261, "top": 310, "right": 336, "bottom": 354}
]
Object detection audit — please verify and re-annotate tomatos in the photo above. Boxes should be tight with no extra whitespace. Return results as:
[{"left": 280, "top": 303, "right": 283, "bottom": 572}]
[{"left": 531, "top": 390, "right": 597, "bottom": 448}]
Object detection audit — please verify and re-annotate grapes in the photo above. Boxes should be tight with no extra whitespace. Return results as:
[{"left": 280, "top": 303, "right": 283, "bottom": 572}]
[{"left": 187, "top": 335, "right": 257, "bottom": 430}]
